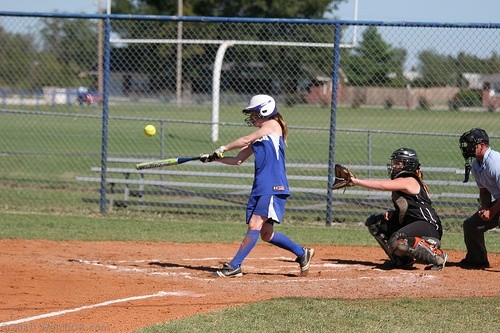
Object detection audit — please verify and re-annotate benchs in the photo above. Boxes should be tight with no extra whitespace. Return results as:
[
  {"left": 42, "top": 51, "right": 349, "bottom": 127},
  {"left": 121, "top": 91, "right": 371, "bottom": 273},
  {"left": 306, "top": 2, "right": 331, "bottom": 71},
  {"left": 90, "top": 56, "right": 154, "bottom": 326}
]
[{"left": 77, "top": 158, "right": 482, "bottom": 211}]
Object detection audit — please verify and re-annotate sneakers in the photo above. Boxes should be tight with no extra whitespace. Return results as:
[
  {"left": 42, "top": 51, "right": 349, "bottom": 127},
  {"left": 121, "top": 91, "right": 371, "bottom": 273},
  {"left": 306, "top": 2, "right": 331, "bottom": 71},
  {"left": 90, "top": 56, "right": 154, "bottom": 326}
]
[
  {"left": 211, "top": 262, "right": 243, "bottom": 279},
  {"left": 296, "top": 248, "right": 314, "bottom": 275}
]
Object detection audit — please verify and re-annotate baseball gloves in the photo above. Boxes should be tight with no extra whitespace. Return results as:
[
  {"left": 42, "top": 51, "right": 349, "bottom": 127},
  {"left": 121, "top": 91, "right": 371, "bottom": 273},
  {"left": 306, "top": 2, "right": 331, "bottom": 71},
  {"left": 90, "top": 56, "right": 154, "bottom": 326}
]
[{"left": 331, "top": 164, "right": 351, "bottom": 190}]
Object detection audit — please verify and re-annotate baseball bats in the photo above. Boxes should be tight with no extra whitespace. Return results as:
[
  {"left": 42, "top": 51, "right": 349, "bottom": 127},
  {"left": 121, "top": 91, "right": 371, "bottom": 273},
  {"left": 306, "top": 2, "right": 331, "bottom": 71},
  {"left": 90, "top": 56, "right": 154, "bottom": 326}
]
[{"left": 136, "top": 152, "right": 224, "bottom": 170}]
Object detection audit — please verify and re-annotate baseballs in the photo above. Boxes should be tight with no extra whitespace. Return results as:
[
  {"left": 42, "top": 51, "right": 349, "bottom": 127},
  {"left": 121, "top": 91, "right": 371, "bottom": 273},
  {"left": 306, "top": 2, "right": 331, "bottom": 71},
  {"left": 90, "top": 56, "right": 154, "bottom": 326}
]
[{"left": 144, "top": 125, "right": 156, "bottom": 137}]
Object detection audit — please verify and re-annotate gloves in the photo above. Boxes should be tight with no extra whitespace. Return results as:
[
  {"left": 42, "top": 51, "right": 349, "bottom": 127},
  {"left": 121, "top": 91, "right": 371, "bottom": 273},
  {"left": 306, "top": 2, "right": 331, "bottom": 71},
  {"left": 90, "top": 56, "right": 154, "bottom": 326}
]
[
  {"left": 213, "top": 149, "right": 224, "bottom": 161},
  {"left": 200, "top": 153, "right": 212, "bottom": 163}
]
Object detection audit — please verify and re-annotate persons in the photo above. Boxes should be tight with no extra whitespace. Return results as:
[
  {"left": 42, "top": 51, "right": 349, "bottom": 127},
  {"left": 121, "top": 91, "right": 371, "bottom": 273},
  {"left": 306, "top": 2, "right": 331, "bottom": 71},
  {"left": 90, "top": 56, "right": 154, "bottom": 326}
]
[
  {"left": 199, "top": 95, "right": 316, "bottom": 278},
  {"left": 459, "top": 128, "right": 500, "bottom": 272},
  {"left": 346, "top": 148, "right": 447, "bottom": 271}
]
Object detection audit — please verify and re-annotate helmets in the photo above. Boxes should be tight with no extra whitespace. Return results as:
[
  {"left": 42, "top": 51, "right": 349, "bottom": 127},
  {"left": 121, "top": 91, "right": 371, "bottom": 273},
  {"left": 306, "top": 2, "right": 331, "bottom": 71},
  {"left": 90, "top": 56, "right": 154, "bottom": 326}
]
[
  {"left": 471, "top": 128, "right": 489, "bottom": 144},
  {"left": 243, "top": 94, "right": 277, "bottom": 118},
  {"left": 391, "top": 148, "right": 417, "bottom": 164}
]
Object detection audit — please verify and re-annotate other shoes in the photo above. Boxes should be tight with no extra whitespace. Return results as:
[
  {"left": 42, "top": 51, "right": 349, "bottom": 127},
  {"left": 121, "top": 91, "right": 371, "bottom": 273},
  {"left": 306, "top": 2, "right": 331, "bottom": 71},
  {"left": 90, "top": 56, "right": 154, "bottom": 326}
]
[
  {"left": 432, "top": 249, "right": 447, "bottom": 270},
  {"left": 460, "top": 259, "right": 489, "bottom": 269},
  {"left": 383, "top": 259, "right": 414, "bottom": 269}
]
